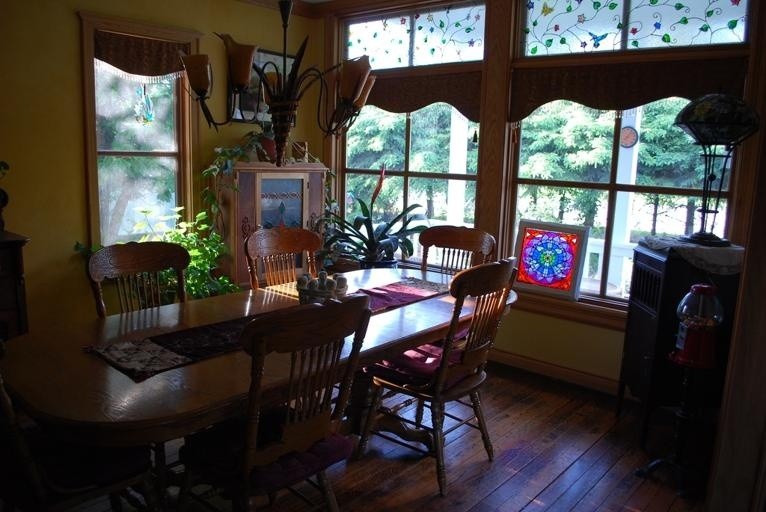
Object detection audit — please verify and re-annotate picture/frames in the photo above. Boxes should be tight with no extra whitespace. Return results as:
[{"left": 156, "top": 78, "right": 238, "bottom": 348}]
[
  {"left": 512, "top": 218, "right": 590, "bottom": 302},
  {"left": 230, "top": 45, "right": 300, "bottom": 129}
]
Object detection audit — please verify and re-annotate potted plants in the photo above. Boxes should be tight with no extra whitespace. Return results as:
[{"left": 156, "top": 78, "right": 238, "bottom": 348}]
[{"left": 202, "top": 131, "right": 339, "bottom": 235}]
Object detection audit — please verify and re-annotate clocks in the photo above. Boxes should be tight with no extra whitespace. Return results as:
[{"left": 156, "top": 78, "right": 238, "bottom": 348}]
[{"left": 619, "top": 126, "right": 639, "bottom": 148}]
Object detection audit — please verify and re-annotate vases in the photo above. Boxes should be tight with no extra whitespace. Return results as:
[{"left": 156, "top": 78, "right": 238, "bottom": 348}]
[{"left": 359, "top": 256, "right": 398, "bottom": 268}]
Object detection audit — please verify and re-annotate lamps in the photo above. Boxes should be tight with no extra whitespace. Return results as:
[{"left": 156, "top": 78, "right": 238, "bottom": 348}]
[
  {"left": 674, "top": 95, "right": 760, "bottom": 247},
  {"left": 179, "top": 2, "right": 375, "bottom": 168}
]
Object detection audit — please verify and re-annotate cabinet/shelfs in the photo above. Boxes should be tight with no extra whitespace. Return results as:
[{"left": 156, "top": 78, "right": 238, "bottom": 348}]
[
  {"left": 613, "top": 248, "right": 744, "bottom": 450},
  {"left": 1, "top": 233, "right": 29, "bottom": 340}
]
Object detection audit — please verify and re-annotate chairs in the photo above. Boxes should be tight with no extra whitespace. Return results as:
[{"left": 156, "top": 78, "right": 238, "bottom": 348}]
[
  {"left": 201, "top": 293, "right": 370, "bottom": 510},
  {"left": 418, "top": 225, "right": 497, "bottom": 273},
  {"left": 86, "top": 241, "right": 190, "bottom": 318},
  {"left": 0, "top": 389, "right": 159, "bottom": 511},
  {"left": 244, "top": 225, "right": 322, "bottom": 288},
  {"left": 348, "top": 256, "right": 517, "bottom": 496}
]
